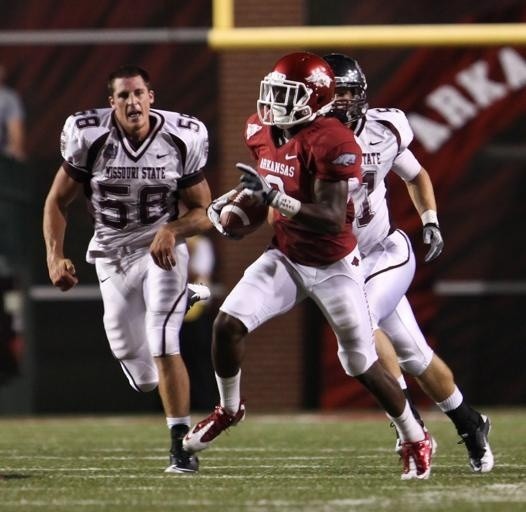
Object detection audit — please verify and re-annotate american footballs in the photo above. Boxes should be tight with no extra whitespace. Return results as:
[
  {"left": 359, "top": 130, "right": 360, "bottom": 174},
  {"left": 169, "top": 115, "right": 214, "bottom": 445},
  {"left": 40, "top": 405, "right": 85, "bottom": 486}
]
[{"left": 220, "top": 189, "right": 267, "bottom": 235}]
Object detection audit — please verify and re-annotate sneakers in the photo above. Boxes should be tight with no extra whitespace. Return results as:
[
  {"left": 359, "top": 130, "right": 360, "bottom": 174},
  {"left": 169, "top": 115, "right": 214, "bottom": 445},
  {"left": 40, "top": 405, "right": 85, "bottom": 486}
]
[
  {"left": 182, "top": 401, "right": 248, "bottom": 454},
  {"left": 460, "top": 413, "right": 495, "bottom": 474},
  {"left": 394, "top": 408, "right": 425, "bottom": 454},
  {"left": 185, "top": 282, "right": 212, "bottom": 315},
  {"left": 162, "top": 422, "right": 199, "bottom": 474},
  {"left": 394, "top": 422, "right": 439, "bottom": 481}
]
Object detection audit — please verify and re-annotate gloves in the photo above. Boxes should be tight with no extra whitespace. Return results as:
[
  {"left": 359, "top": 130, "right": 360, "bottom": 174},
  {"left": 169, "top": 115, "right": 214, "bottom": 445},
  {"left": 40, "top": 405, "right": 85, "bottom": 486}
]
[
  {"left": 233, "top": 161, "right": 300, "bottom": 221},
  {"left": 205, "top": 189, "right": 239, "bottom": 240},
  {"left": 421, "top": 209, "right": 446, "bottom": 264}
]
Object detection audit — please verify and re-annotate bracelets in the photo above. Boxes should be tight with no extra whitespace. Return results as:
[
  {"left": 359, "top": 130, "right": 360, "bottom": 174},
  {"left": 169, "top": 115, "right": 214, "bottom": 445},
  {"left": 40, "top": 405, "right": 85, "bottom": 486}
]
[
  {"left": 418, "top": 208, "right": 440, "bottom": 229},
  {"left": 223, "top": 188, "right": 239, "bottom": 200},
  {"left": 270, "top": 191, "right": 301, "bottom": 219}
]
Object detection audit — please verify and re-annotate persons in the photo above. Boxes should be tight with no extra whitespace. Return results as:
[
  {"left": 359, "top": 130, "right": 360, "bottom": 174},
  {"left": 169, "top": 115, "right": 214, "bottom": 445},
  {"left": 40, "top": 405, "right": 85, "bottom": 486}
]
[
  {"left": 1, "top": 86, "right": 27, "bottom": 162},
  {"left": 42, "top": 68, "right": 217, "bottom": 472},
  {"left": 180, "top": 51, "right": 438, "bottom": 478},
  {"left": 177, "top": 201, "right": 217, "bottom": 283},
  {"left": 267, "top": 52, "right": 495, "bottom": 472}
]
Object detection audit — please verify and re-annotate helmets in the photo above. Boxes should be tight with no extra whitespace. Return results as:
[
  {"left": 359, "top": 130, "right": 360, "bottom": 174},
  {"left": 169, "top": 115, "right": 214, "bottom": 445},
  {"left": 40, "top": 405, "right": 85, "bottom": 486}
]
[
  {"left": 256, "top": 52, "right": 337, "bottom": 130},
  {"left": 322, "top": 54, "right": 370, "bottom": 126}
]
